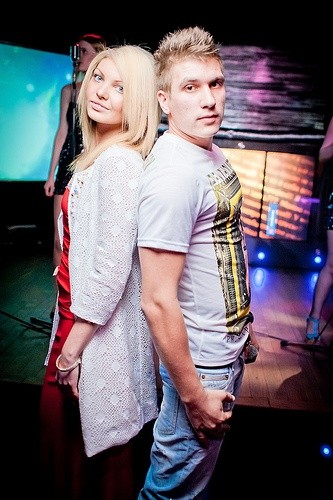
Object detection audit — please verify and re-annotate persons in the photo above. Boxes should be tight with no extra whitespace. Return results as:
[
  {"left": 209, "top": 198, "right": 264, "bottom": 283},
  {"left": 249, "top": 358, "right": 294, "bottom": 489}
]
[
  {"left": 136, "top": 26, "right": 259, "bottom": 500},
  {"left": 45, "top": 34, "right": 105, "bottom": 289},
  {"left": 306, "top": 117, "right": 333, "bottom": 345},
  {"left": 40, "top": 46, "right": 162, "bottom": 500}
]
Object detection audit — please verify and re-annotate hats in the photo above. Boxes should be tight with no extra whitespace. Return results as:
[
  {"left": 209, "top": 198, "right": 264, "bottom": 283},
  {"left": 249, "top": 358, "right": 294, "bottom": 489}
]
[{"left": 82, "top": 34, "right": 104, "bottom": 52}]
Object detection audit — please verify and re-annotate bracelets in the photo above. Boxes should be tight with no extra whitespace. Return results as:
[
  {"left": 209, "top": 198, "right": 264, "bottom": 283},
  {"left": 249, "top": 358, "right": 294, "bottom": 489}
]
[{"left": 56, "top": 354, "right": 81, "bottom": 372}]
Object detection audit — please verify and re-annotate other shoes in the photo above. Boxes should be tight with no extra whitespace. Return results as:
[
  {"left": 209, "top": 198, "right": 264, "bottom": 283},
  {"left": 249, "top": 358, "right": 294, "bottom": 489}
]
[
  {"left": 305, "top": 315, "right": 320, "bottom": 345},
  {"left": 50, "top": 305, "right": 55, "bottom": 319}
]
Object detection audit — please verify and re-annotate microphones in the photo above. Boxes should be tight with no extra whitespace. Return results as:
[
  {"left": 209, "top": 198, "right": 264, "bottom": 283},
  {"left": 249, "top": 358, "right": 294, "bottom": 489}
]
[
  {"left": 70, "top": 43, "right": 81, "bottom": 61},
  {"left": 244, "top": 344, "right": 258, "bottom": 364}
]
